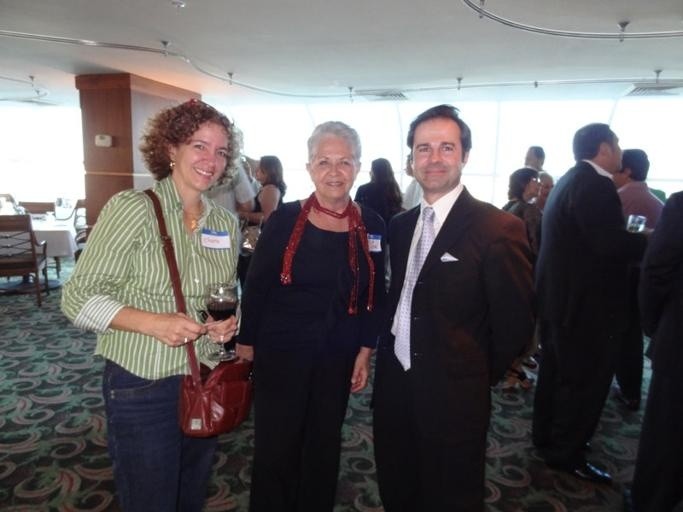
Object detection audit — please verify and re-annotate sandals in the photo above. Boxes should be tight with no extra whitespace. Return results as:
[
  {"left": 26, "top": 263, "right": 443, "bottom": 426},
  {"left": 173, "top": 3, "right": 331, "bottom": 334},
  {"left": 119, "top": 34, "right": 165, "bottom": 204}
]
[{"left": 506, "top": 369, "right": 536, "bottom": 388}]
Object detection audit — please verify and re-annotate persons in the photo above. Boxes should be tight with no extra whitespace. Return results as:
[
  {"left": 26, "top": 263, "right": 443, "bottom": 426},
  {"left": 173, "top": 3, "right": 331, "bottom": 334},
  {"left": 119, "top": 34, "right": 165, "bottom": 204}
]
[
  {"left": 503, "top": 166, "right": 545, "bottom": 390},
  {"left": 534, "top": 122, "right": 656, "bottom": 485},
  {"left": 204, "top": 160, "right": 256, "bottom": 216},
  {"left": 59, "top": 100, "right": 244, "bottom": 511},
  {"left": 245, "top": 156, "right": 288, "bottom": 226},
  {"left": 610, "top": 150, "right": 667, "bottom": 409},
  {"left": 236, "top": 124, "right": 386, "bottom": 511},
  {"left": 521, "top": 146, "right": 547, "bottom": 182},
  {"left": 632, "top": 187, "right": 682, "bottom": 510},
  {"left": 401, "top": 153, "right": 424, "bottom": 210},
  {"left": 529, "top": 172, "right": 554, "bottom": 241},
  {"left": 371, "top": 105, "right": 533, "bottom": 512},
  {"left": 358, "top": 159, "right": 403, "bottom": 220}
]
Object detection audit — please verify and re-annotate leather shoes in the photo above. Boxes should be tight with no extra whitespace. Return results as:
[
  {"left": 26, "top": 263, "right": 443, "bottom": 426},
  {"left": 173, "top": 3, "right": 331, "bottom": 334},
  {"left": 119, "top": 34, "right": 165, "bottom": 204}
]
[
  {"left": 614, "top": 384, "right": 641, "bottom": 409},
  {"left": 625, "top": 494, "right": 637, "bottom": 511},
  {"left": 575, "top": 462, "right": 615, "bottom": 484}
]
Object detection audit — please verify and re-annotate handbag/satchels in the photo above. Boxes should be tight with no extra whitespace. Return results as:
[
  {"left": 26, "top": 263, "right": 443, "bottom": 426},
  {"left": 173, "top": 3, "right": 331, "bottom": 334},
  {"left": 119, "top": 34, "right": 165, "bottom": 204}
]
[
  {"left": 241, "top": 213, "right": 263, "bottom": 257},
  {"left": 144, "top": 188, "right": 254, "bottom": 437}
]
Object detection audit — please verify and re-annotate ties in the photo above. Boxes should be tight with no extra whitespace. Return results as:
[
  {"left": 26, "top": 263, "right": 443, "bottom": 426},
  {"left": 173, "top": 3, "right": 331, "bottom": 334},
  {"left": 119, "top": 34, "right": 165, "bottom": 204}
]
[{"left": 395, "top": 208, "right": 437, "bottom": 372}]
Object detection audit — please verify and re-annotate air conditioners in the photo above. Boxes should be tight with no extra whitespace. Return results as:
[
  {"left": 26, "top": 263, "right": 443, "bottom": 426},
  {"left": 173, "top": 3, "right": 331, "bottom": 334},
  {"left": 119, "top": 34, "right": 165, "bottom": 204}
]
[
  {"left": 355, "top": 87, "right": 407, "bottom": 104},
  {"left": 623, "top": 82, "right": 682, "bottom": 98}
]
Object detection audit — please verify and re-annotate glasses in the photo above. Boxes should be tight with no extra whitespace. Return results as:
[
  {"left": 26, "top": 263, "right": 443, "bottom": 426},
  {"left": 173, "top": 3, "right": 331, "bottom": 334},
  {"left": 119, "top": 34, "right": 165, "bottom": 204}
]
[{"left": 531, "top": 176, "right": 542, "bottom": 182}]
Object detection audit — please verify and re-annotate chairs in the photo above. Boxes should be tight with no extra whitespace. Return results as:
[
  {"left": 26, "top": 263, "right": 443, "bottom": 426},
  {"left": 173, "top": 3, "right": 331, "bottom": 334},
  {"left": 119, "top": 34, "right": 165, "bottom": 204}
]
[
  {"left": 17, "top": 201, "right": 62, "bottom": 278},
  {"left": 0, "top": 214, "right": 50, "bottom": 308},
  {"left": 72, "top": 198, "right": 94, "bottom": 264}
]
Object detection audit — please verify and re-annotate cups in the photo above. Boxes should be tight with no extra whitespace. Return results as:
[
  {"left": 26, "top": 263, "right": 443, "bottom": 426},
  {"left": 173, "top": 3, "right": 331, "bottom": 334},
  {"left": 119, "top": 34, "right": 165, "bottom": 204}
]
[
  {"left": 625, "top": 213, "right": 647, "bottom": 234},
  {"left": 205, "top": 283, "right": 239, "bottom": 362}
]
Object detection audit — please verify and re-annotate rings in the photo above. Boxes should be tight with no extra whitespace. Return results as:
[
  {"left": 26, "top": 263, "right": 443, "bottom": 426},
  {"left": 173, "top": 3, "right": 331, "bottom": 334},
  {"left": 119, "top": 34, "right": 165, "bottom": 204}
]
[
  {"left": 185, "top": 335, "right": 188, "bottom": 345},
  {"left": 219, "top": 336, "right": 226, "bottom": 345}
]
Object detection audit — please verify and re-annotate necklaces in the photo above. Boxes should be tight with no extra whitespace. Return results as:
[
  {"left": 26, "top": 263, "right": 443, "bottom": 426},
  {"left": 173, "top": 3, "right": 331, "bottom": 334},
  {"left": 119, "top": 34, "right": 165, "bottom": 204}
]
[{"left": 184, "top": 208, "right": 204, "bottom": 231}]
